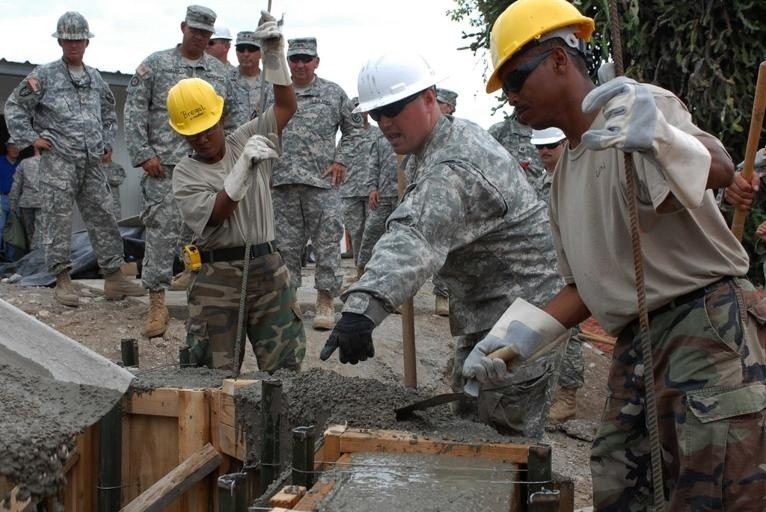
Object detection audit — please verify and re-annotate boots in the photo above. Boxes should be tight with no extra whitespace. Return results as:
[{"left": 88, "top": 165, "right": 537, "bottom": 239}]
[
  {"left": 435, "top": 294, "right": 449, "bottom": 315},
  {"left": 53, "top": 269, "right": 79, "bottom": 306},
  {"left": 104, "top": 268, "right": 149, "bottom": 299},
  {"left": 168, "top": 266, "right": 198, "bottom": 290},
  {"left": 544, "top": 384, "right": 577, "bottom": 430},
  {"left": 313, "top": 290, "right": 336, "bottom": 330},
  {"left": 145, "top": 289, "right": 170, "bottom": 338}
]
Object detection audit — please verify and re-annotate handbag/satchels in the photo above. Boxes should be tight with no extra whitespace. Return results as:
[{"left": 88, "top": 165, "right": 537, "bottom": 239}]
[{"left": 4, "top": 211, "right": 27, "bottom": 251}]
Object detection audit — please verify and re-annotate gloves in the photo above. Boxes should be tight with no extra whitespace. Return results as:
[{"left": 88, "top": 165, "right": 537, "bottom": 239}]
[
  {"left": 253, "top": 8, "right": 293, "bottom": 86},
  {"left": 581, "top": 62, "right": 712, "bottom": 209},
  {"left": 320, "top": 313, "right": 375, "bottom": 365},
  {"left": 224, "top": 133, "right": 280, "bottom": 202},
  {"left": 462, "top": 296, "right": 569, "bottom": 401}
]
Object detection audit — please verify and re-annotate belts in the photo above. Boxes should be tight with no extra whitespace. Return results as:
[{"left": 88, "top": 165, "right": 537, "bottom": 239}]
[
  {"left": 618, "top": 275, "right": 729, "bottom": 342},
  {"left": 199, "top": 239, "right": 276, "bottom": 264}
]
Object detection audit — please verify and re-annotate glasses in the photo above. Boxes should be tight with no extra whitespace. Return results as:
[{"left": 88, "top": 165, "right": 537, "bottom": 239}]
[
  {"left": 367, "top": 89, "right": 429, "bottom": 122},
  {"left": 185, "top": 20, "right": 213, "bottom": 38},
  {"left": 235, "top": 44, "right": 260, "bottom": 52},
  {"left": 68, "top": 69, "right": 92, "bottom": 88},
  {"left": 500, "top": 48, "right": 577, "bottom": 96},
  {"left": 289, "top": 54, "right": 317, "bottom": 63},
  {"left": 181, "top": 120, "right": 223, "bottom": 142},
  {"left": 535, "top": 138, "right": 568, "bottom": 150}
]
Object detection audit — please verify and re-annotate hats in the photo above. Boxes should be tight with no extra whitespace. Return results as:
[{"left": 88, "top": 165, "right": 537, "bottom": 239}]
[
  {"left": 352, "top": 97, "right": 358, "bottom": 103},
  {"left": 436, "top": 87, "right": 459, "bottom": 106},
  {"left": 287, "top": 38, "right": 316, "bottom": 56},
  {"left": 236, "top": 31, "right": 260, "bottom": 47},
  {"left": 184, "top": 5, "right": 216, "bottom": 32}
]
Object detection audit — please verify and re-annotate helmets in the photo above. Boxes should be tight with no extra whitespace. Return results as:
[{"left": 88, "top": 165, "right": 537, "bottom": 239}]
[
  {"left": 5, "top": 136, "right": 16, "bottom": 144},
  {"left": 51, "top": 12, "right": 95, "bottom": 40},
  {"left": 209, "top": 25, "right": 233, "bottom": 40},
  {"left": 487, "top": 0, "right": 596, "bottom": 94},
  {"left": 167, "top": 77, "right": 225, "bottom": 136}
]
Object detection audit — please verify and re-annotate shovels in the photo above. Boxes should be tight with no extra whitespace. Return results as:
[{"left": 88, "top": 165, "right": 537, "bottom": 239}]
[{"left": 394, "top": 343, "right": 519, "bottom": 413}]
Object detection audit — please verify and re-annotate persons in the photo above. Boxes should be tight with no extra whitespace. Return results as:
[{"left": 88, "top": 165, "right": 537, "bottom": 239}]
[
  {"left": 715, "top": 147, "right": 766, "bottom": 282},
  {"left": 455, "top": 1, "right": 765, "bottom": 512},
  {"left": 338, "top": 87, "right": 546, "bottom": 313},
  {"left": 122, "top": 2, "right": 365, "bottom": 375},
  {"left": 526, "top": 127, "right": 586, "bottom": 432},
  {"left": 318, "top": 48, "right": 573, "bottom": 443},
  {"left": 0, "top": 11, "right": 146, "bottom": 305}
]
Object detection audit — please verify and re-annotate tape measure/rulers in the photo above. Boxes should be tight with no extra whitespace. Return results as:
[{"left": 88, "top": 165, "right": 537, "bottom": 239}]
[{"left": 181, "top": 245, "right": 203, "bottom": 272}]
